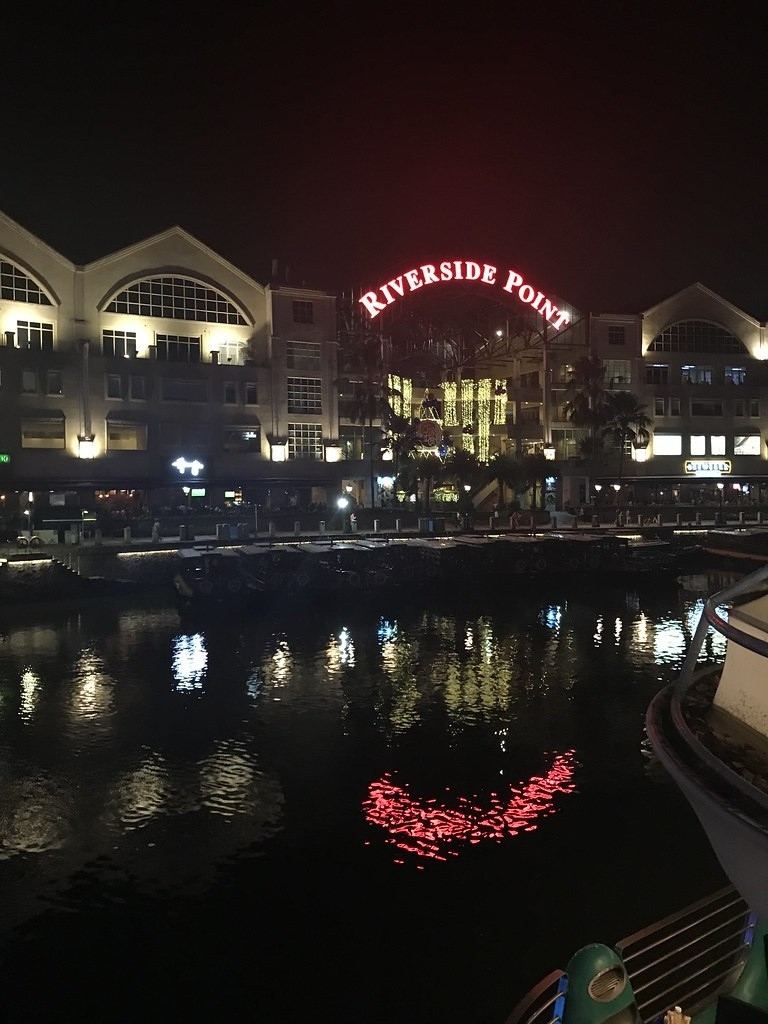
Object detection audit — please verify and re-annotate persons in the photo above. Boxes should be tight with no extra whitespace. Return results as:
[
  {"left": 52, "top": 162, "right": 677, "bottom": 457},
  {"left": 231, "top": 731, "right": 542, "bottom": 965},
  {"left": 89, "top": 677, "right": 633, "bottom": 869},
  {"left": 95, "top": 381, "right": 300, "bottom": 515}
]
[
  {"left": 455, "top": 510, "right": 472, "bottom": 530},
  {"left": 350, "top": 511, "right": 356, "bottom": 522},
  {"left": 492, "top": 501, "right": 522, "bottom": 529}
]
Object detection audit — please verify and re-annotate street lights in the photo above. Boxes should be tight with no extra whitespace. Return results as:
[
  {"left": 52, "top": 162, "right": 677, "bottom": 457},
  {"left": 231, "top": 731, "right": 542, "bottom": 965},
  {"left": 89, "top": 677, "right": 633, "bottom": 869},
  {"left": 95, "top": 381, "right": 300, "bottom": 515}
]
[
  {"left": 464, "top": 484, "right": 473, "bottom": 530},
  {"left": 717, "top": 482, "right": 724, "bottom": 524},
  {"left": 614, "top": 484, "right": 622, "bottom": 527},
  {"left": 595, "top": 484, "right": 603, "bottom": 528},
  {"left": 181, "top": 486, "right": 193, "bottom": 540}
]
